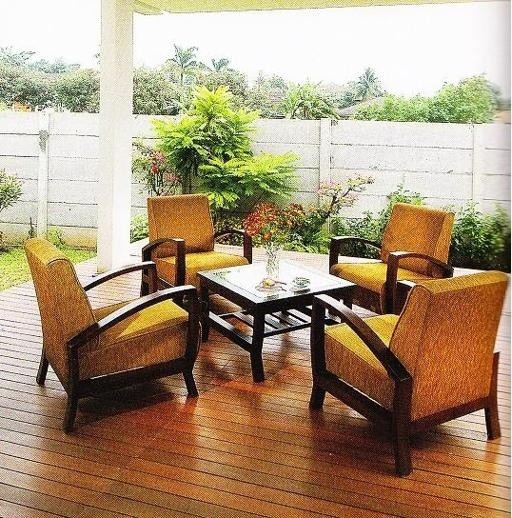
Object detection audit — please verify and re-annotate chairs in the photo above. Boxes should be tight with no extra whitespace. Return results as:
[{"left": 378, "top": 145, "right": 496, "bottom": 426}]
[
  {"left": 309, "top": 270, "right": 509, "bottom": 476},
  {"left": 142, "top": 195, "right": 252, "bottom": 319},
  {"left": 22, "top": 238, "right": 203, "bottom": 432},
  {"left": 328, "top": 202, "right": 453, "bottom": 317}
]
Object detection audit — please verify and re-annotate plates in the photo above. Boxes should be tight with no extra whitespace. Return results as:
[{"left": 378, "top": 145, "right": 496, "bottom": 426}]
[{"left": 290, "top": 286, "right": 310, "bottom": 292}]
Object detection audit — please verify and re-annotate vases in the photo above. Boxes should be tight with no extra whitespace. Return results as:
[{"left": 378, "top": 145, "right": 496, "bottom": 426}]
[{"left": 263, "top": 244, "right": 284, "bottom": 278}]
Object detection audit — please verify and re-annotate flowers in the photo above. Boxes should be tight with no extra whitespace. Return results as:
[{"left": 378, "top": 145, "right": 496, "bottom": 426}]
[{"left": 243, "top": 200, "right": 306, "bottom": 272}]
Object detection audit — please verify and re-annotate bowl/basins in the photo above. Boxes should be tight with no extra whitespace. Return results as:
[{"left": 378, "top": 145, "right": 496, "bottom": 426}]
[{"left": 292, "top": 276, "right": 310, "bottom": 287}]
